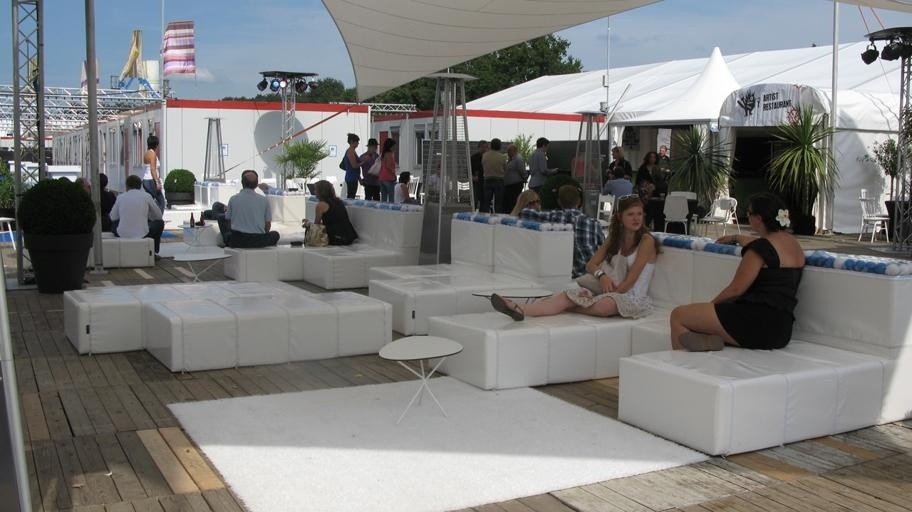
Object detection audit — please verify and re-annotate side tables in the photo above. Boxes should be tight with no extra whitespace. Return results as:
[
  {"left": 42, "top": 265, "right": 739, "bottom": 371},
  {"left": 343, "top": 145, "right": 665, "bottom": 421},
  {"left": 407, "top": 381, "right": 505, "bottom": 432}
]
[
  {"left": 178, "top": 224, "right": 212, "bottom": 253},
  {"left": 472, "top": 288, "right": 554, "bottom": 305},
  {"left": 379, "top": 337, "right": 464, "bottom": 422},
  {"left": 174, "top": 254, "right": 231, "bottom": 282}
]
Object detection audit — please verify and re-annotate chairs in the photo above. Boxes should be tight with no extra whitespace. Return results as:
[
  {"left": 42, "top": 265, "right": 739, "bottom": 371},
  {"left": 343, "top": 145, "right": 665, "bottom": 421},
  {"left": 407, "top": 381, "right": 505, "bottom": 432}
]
[
  {"left": 859, "top": 189, "right": 891, "bottom": 243},
  {"left": 598, "top": 191, "right": 742, "bottom": 237},
  {"left": 397, "top": 174, "right": 470, "bottom": 202}
]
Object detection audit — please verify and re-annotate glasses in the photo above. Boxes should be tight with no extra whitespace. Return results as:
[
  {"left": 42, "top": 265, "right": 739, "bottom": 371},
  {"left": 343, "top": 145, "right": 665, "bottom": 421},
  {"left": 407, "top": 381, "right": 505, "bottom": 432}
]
[{"left": 617, "top": 194, "right": 640, "bottom": 211}]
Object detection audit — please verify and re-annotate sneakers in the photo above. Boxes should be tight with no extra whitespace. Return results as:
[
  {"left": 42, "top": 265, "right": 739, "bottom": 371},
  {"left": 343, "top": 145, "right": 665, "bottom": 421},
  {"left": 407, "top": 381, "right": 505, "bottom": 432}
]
[{"left": 679, "top": 332, "right": 724, "bottom": 351}]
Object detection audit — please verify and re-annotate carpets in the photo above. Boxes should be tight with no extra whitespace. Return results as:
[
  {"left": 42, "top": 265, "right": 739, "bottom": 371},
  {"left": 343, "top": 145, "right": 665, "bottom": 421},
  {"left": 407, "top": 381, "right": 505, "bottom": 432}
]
[
  {"left": 167, "top": 375, "right": 711, "bottom": 512},
  {"left": 155, "top": 242, "right": 190, "bottom": 257}
]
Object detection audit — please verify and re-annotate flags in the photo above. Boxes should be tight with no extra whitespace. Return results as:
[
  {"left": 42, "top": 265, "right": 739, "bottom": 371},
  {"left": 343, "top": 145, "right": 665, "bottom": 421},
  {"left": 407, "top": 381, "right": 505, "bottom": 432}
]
[
  {"left": 159, "top": 19, "right": 196, "bottom": 78},
  {"left": 118, "top": 30, "right": 141, "bottom": 81}
]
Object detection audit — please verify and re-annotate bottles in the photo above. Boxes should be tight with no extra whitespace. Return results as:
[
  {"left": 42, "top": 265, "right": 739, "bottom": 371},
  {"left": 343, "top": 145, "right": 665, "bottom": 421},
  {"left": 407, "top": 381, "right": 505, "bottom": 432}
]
[
  {"left": 189, "top": 212, "right": 195, "bottom": 227},
  {"left": 200, "top": 212, "right": 204, "bottom": 226}
]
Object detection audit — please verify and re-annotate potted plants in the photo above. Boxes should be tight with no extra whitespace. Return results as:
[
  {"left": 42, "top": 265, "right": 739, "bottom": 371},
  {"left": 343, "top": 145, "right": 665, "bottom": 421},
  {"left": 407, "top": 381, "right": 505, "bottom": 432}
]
[
  {"left": 18, "top": 178, "right": 95, "bottom": 295},
  {"left": 164, "top": 169, "right": 194, "bottom": 209},
  {"left": 763, "top": 99, "right": 843, "bottom": 236},
  {"left": 0, "top": 178, "right": 16, "bottom": 216}
]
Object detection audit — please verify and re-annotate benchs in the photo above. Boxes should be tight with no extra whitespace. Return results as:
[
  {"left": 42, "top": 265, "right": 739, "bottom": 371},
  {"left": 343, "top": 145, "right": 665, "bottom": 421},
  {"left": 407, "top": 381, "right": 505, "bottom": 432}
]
[{"left": 87, "top": 232, "right": 156, "bottom": 267}]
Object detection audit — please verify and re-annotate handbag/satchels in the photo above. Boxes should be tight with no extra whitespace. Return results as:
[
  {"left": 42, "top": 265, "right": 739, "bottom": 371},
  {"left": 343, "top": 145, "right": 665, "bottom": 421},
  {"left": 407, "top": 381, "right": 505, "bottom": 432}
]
[
  {"left": 304, "top": 224, "right": 329, "bottom": 247},
  {"left": 368, "top": 153, "right": 382, "bottom": 176},
  {"left": 339, "top": 153, "right": 351, "bottom": 170}
]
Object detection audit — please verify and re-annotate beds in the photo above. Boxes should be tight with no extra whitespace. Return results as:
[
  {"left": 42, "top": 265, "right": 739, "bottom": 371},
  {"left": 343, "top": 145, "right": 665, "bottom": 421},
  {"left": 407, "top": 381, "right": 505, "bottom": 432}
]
[
  {"left": 430, "top": 231, "right": 707, "bottom": 388},
  {"left": 367, "top": 211, "right": 575, "bottom": 334},
  {"left": 618, "top": 229, "right": 912, "bottom": 458},
  {"left": 225, "top": 195, "right": 424, "bottom": 289},
  {"left": 160, "top": 182, "right": 240, "bottom": 243}
]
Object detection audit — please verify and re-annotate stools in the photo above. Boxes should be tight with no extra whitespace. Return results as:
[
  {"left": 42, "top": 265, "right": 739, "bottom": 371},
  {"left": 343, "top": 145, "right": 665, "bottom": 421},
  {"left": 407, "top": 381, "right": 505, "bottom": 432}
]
[{"left": 0, "top": 218, "right": 17, "bottom": 251}]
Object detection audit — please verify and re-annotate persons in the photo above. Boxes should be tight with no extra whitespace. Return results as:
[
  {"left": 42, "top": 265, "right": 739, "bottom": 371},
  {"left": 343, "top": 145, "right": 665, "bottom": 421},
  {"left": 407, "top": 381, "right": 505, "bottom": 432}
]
[
  {"left": 143, "top": 136, "right": 165, "bottom": 216},
  {"left": 226, "top": 170, "right": 280, "bottom": 247},
  {"left": 466, "top": 137, "right": 670, "bottom": 231},
  {"left": 76, "top": 177, "right": 91, "bottom": 199},
  {"left": 100, "top": 173, "right": 120, "bottom": 237},
  {"left": 671, "top": 192, "right": 805, "bottom": 351},
  {"left": 344, "top": 133, "right": 451, "bottom": 204},
  {"left": 302, "top": 180, "right": 358, "bottom": 246},
  {"left": 521, "top": 185, "right": 606, "bottom": 280},
  {"left": 491, "top": 193, "right": 659, "bottom": 319},
  {"left": 108, "top": 175, "right": 164, "bottom": 256}
]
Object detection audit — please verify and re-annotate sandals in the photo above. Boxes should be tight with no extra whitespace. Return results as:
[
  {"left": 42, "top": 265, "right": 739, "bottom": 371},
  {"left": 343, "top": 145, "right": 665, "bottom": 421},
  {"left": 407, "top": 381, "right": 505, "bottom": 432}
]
[{"left": 491, "top": 294, "right": 524, "bottom": 321}]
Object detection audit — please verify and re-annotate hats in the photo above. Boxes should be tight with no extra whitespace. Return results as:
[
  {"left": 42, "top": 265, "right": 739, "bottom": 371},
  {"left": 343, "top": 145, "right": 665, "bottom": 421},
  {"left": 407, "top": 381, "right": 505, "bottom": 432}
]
[{"left": 366, "top": 139, "right": 379, "bottom": 146}]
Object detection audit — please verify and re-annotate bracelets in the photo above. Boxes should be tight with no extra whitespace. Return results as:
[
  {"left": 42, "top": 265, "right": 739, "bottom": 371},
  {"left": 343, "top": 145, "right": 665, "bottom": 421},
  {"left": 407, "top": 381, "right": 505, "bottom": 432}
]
[{"left": 593, "top": 269, "right": 604, "bottom": 279}]
